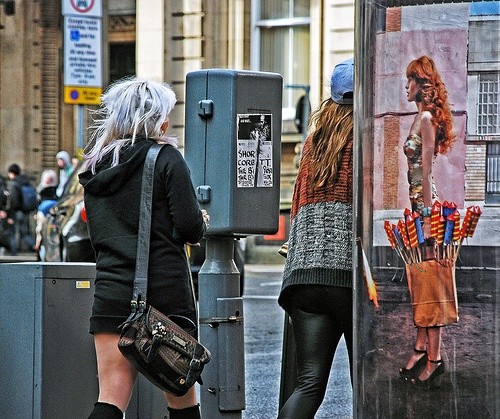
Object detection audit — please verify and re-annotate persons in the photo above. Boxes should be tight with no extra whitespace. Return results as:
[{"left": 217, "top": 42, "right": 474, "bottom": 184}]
[
  {"left": 0, "top": 151, "right": 80, "bottom": 256},
  {"left": 276, "top": 58, "right": 353, "bottom": 419},
  {"left": 78, "top": 74, "right": 211, "bottom": 419}
]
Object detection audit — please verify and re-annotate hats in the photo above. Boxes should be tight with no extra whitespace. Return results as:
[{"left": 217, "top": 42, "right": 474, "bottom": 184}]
[{"left": 331, "top": 58, "right": 353, "bottom": 104}]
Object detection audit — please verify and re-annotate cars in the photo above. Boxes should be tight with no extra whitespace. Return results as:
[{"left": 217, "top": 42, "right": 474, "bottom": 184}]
[{"left": 36, "top": 147, "right": 246, "bottom": 302}]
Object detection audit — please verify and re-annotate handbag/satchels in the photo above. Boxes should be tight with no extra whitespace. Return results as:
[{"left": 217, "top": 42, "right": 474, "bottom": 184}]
[{"left": 117, "top": 306, "right": 212, "bottom": 396}]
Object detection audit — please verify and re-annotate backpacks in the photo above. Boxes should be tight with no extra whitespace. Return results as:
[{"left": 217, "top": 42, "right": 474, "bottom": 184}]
[{"left": 14, "top": 179, "right": 37, "bottom": 209}]
